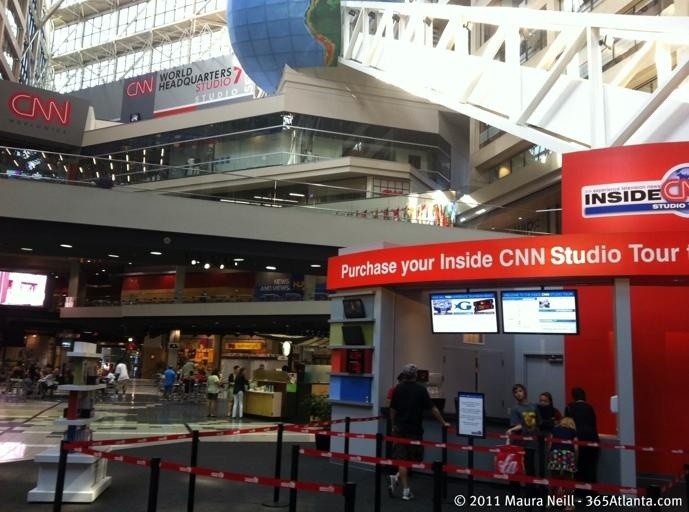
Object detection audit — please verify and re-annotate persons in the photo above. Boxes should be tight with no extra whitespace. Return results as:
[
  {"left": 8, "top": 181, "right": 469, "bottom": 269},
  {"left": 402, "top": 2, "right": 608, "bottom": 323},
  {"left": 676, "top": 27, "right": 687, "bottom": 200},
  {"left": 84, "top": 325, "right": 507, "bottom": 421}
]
[
  {"left": 226, "top": 364, "right": 240, "bottom": 417},
  {"left": 385, "top": 372, "right": 403, "bottom": 410},
  {"left": 538, "top": 391, "right": 562, "bottom": 432},
  {"left": 231, "top": 367, "right": 252, "bottom": 419},
  {"left": 205, "top": 367, "right": 223, "bottom": 418},
  {"left": 509, "top": 384, "right": 543, "bottom": 490},
  {"left": 387, "top": 364, "right": 451, "bottom": 500},
  {"left": 85, "top": 356, "right": 130, "bottom": 402},
  {"left": 547, "top": 417, "right": 579, "bottom": 498},
  {"left": 0, "top": 359, "right": 69, "bottom": 400},
  {"left": 185, "top": 156, "right": 200, "bottom": 178},
  {"left": 154, "top": 360, "right": 211, "bottom": 404},
  {"left": 564, "top": 387, "right": 599, "bottom": 503}
]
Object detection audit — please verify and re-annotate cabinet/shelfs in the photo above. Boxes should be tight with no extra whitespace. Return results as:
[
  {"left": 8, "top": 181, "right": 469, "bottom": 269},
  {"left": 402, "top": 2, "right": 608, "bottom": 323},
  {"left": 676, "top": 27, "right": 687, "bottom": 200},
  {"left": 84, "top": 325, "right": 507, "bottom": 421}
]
[{"left": 244, "top": 390, "right": 282, "bottom": 417}]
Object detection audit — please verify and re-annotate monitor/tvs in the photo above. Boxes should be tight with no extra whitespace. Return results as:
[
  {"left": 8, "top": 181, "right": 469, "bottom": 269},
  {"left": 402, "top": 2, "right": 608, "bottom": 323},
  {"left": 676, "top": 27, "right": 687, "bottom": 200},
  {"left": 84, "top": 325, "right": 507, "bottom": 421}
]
[
  {"left": 429, "top": 290, "right": 500, "bottom": 334},
  {"left": 0, "top": 267, "right": 55, "bottom": 310},
  {"left": 500, "top": 290, "right": 580, "bottom": 335}
]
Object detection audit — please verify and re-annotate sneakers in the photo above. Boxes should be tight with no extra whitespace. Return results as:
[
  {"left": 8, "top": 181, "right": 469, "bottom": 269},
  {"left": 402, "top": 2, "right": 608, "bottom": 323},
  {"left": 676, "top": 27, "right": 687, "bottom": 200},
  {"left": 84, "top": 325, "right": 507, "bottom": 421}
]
[
  {"left": 387, "top": 475, "right": 398, "bottom": 498},
  {"left": 402, "top": 489, "right": 414, "bottom": 500}
]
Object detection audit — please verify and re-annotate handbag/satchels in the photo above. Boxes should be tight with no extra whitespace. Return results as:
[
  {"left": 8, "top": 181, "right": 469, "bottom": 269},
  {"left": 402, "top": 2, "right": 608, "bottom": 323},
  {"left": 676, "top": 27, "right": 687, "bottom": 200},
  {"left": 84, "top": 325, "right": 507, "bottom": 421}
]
[{"left": 494, "top": 437, "right": 527, "bottom": 475}]
